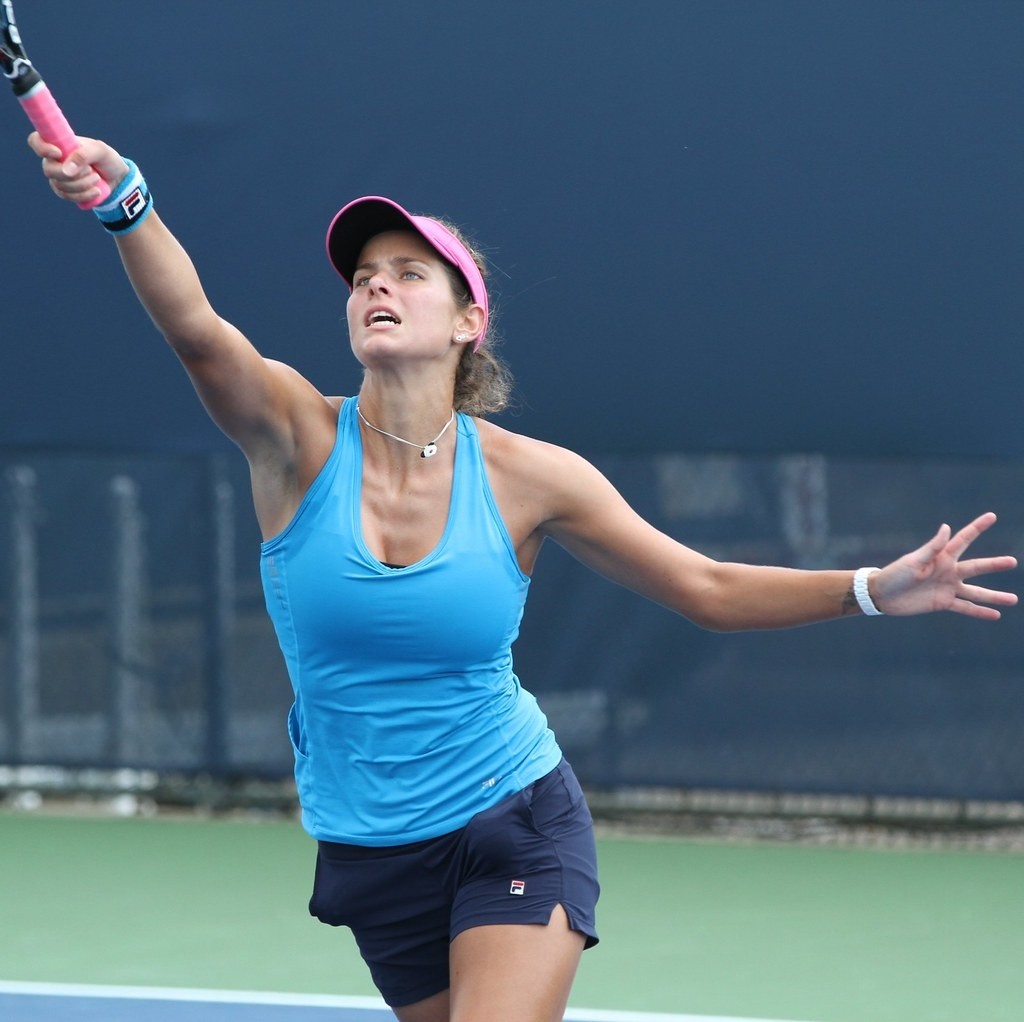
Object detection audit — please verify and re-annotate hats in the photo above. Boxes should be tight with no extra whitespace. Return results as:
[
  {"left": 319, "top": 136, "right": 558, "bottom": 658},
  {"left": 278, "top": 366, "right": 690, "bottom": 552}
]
[{"left": 326, "top": 195, "right": 490, "bottom": 352}]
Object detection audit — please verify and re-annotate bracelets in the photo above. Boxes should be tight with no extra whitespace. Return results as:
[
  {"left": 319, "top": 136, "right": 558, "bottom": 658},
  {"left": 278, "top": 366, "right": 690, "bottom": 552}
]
[
  {"left": 853, "top": 566, "right": 883, "bottom": 616},
  {"left": 91, "top": 157, "right": 154, "bottom": 235}
]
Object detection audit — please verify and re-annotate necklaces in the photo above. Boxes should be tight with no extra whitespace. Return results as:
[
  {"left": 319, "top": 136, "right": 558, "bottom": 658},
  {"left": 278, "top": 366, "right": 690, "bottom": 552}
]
[{"left": 355, "top": 403, "right": 455, "bottom": 458}]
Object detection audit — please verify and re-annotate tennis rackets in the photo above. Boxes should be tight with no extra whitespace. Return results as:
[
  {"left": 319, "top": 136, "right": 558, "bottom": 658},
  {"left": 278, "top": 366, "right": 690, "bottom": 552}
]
[{"left": 0, "top": 0, "right": 112, "bottom": 212}]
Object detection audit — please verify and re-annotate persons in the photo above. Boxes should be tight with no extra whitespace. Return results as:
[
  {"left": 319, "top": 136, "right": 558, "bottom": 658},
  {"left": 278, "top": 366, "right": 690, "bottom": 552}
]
[{"left": 23, "top": 128, "right": 1018, "bottom": 1022}]
